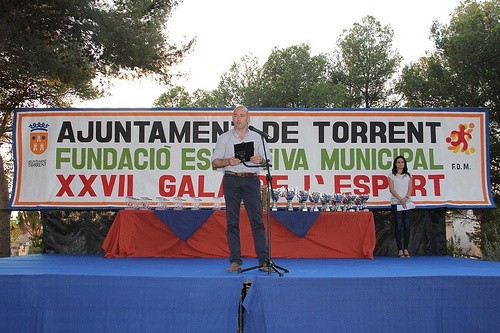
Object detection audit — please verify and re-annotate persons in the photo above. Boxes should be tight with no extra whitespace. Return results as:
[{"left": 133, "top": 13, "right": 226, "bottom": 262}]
[
  {"left": 388, "top": 156, "right": 412, "bottom": 258},
  {"left": 212, "top": 106, "right": 278, "bottom": 273}
]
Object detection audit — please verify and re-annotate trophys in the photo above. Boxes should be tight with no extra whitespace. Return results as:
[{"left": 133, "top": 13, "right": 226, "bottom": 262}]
[
  {"left": 296, "top": 190, "right": 309, "bottom": 211},
  {"left": 342, "top": 194, "right": 356, "bottom": 212},
  {"left": 269, "top": 187, "right": 283, "bottom": 211},
  {"left": 320, "top": 193, "right": 333, "bottom": 211},
  {"left": 284, "top": 187, "right": 295, "bottom": 210},
  {"left": 330, "top": 193, "right": 344, "bottom": 211},
  {"left": 355, "top": 194, "right": 370, "bottom": 212},
  {"left": 309, "top": 191, "right": 321, "bottom": 212}
]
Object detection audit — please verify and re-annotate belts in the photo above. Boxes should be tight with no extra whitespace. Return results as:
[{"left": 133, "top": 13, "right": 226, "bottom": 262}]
[{"left": 224, "top": 171, "right": 257, "bottom": 177}]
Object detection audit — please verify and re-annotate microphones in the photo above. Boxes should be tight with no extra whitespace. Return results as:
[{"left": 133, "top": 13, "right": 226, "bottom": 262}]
[{"left": 248, "top": 125, "right": 269, "bottom": 139}]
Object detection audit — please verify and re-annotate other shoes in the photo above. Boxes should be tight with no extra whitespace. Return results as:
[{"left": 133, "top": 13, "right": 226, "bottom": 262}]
[
  {"left": 398, "top": 254, "right": 405, "bottom": 258},
  {"left": 259, "top": 266, "right": 276, "bottom": 273},
  {"left": 404, "top": 253, "right": 410, "bottom": 257},
  {"left": 227, "top": 262, "right": 241, "bottom": 271}
]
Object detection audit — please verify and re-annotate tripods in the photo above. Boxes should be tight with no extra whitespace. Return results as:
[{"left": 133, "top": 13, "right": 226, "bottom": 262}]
[{"left": 237, "top": 137, "right": 290, "bottom": 276}]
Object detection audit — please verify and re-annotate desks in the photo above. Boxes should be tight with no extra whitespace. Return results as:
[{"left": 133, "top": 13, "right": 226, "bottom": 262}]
[{"left": 103, "top": 209, "right": 377, "bottom": 258}]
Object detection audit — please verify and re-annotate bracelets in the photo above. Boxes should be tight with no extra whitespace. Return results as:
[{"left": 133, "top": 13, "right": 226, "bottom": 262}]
[{"left": 406, "top": 196, "right": 409, "bottom": 199}]
